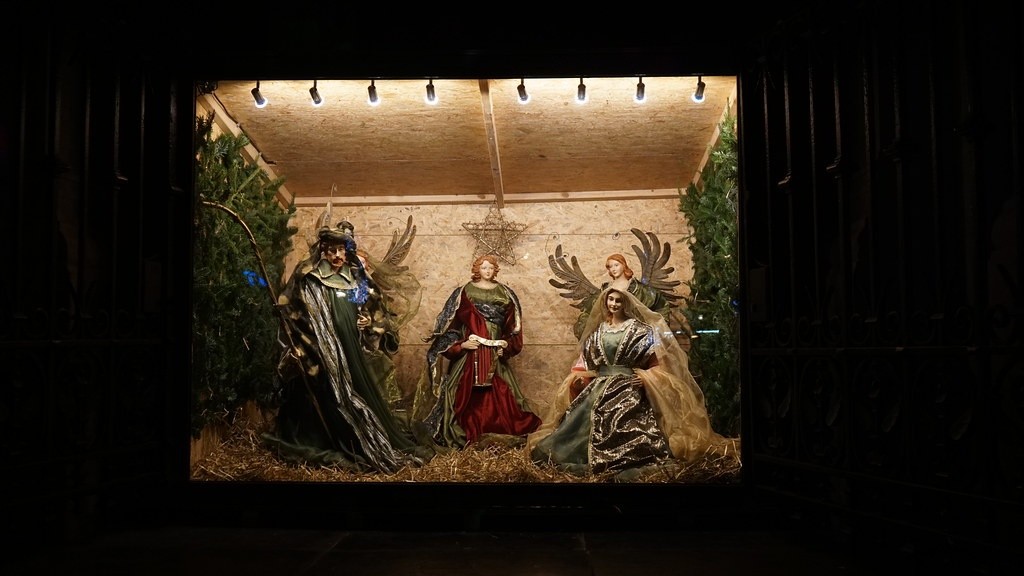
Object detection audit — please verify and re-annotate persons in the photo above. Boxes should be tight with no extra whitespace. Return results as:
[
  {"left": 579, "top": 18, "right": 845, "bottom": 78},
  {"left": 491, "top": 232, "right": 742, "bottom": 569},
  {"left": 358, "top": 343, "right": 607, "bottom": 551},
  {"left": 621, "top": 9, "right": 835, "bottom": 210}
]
[
  {"left": 264, "top": 220, "right": 434, "bottom": 473},
  {"left": 601, "top": 255, "right": 669, "bottom": 328},
  {"left": 527, "top": 287, "right": 732, "bottom": 474},
  {"left": 409, "top": 255, "right": 542, "bottom": 446}
]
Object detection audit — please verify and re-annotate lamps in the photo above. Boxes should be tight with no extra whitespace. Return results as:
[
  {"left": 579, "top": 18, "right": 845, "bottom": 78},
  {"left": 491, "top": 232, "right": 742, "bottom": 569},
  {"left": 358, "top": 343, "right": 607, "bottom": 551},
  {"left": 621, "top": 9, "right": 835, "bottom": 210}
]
[
  {"left": 368, "top": 80, "right": 380, "bottom": 106},
  {"left": 633, "top": 77, "right": 647, "bottom": 103},
  {"left": 576, "top": 78, "right": 588, "bottom": 105},
  {"left": 691, "top": 77, "right": 706, "bottom": 103},
  {"left": 516, "top": 79, "right": 532, "bottom": 104},
  {"left": 425, "top": 80, "right": 438, "bottom": 104},
  {"left": 250, "top": 80, "right": 269, "bottom": 108},
  {"left": 309, "top": 80, "right": 324, "bottom": 107}
]
